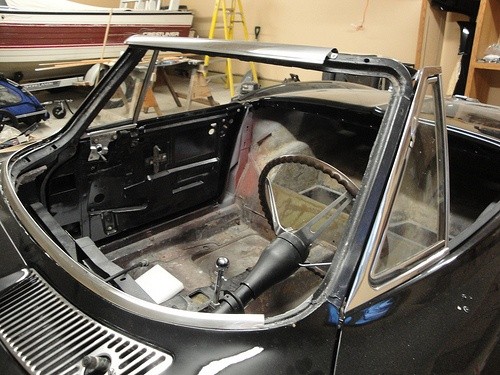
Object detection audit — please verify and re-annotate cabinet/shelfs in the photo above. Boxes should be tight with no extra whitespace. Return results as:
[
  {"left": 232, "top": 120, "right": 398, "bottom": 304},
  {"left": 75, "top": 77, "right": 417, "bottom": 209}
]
[{"left": 462, "top": 0, "right": 500, "bottom": 109}]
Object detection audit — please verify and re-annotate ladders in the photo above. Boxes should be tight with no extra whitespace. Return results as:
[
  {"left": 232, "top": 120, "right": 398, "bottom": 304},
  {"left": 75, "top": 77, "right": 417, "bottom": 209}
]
[{"left": 198, "top": 0, "right": 260, "bottom": 101}]
